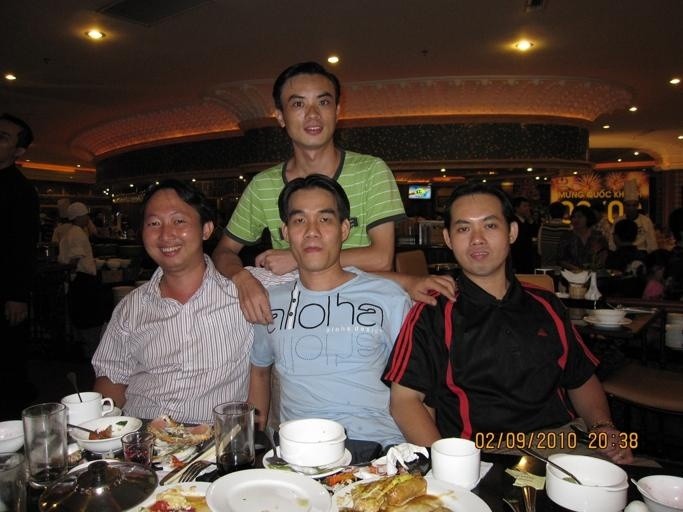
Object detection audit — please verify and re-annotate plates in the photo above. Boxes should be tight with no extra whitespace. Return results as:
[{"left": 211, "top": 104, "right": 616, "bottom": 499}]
[
  {"left": 206, "top": 469, "right": 331, "bottom": 511},
  {"left": 263, "top": 445, "right": 353, "bottom": 478},
  {"left": 128, "top": 481, "right": 211, "bottom": 512},
  {"left": 51, "top": 404, "right": 122, "bottom": 433},
  {"left": 331, "top": 478, "right": 492, "bottom": 512},
  {"left": 583, "top": 315, "right": 631, "bottom": 328}
]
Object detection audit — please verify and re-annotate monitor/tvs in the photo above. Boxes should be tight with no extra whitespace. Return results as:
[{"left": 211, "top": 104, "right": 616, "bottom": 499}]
[{"left": 407, "top": 184, "right": 433, "bottom": 201}]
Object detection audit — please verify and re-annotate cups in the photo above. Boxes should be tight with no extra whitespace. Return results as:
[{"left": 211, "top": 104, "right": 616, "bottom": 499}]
[
  {"left": 121, "top": 431, "right": 153, "bottom": 467},
  {"left": 431, "top": 438, "right": 480, "bottom": 486},
  {"left": 61, "top": 392, "right": 113, "bottom": 426},
  {"left": 21, "top": 402, "right": 68, "bottom": 491},
  {"left": 214, "top": 400, "right": 256, "bottom": 470},
  {"left": 0, "top": 450, "right": 29, "bottom": 511}
]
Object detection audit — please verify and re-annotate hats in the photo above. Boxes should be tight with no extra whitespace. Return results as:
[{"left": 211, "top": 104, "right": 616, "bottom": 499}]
[
  {"left": 67, "top": 202, "right": 93, "bottom": 222},
  {"left": 54, "top": 199, "right": 71, "bottom": 218}
]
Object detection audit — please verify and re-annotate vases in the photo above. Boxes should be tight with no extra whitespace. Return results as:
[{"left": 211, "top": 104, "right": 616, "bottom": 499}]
[
  {"left": 584, "top": 271, "right": 603, "bottom": 301},
  {"left": 560, "top": 267, "right": 589, "bottom": 286}
]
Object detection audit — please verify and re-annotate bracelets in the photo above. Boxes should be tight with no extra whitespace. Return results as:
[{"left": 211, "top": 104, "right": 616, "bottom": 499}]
[{"left": 592, "top": 418, "right": 616, "bottom": 429}]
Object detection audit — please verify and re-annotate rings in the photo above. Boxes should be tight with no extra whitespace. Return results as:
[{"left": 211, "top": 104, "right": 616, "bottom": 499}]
[{"left": 618, "top": 449, "right": 626, "bottom": 460}]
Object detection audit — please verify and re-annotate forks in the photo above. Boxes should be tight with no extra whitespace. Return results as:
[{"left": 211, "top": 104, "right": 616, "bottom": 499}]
[{"left": 178, "top": 459, "right": 215, "bottom": 483}]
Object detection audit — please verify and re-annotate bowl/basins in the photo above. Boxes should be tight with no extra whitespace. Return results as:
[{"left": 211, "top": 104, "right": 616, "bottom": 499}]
[
  {"left": 70, "top": 415, "right": 143, "bottom": 457},
  {"left": 0, "top": 419, "right": 25, "bottom": 456},
  {"left": 637, "top": 475, "right": 683, "bottom": 512},
  {"left": 277, "top": 417, "right": 346, "bottom": 466},
  {"left": 593, "top": 309, "right": 626, "bottom": 322},
  {"left": 545, "top": 452, "right": 630, "bottom": 512},
  {"left": 121, "top": 260, "right": 131, "bottom": 267},
  {"left": 95, "top": 260, "right": 105, "bottom": 271},
  {"left": 107, "top": 261, "right": 121, "bottom": 271}
]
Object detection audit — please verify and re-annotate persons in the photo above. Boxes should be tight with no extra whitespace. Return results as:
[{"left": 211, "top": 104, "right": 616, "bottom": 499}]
[
  {"left": 61, "top": 202, "right": 96, "bottom": 280},
  {"left": 605, "top": 177, "right": 657, "bottom": 252},
  {"left": 559, "top": 204, "right": 608, "bottom": 268},
  {"left": 538, "top": 201, "right": 577, "bottom": 268},
  {"left": 510, "top": 195, "right": 533, "bottom": 244},
  {"left": 52, "top": 198, "right": 71, "bottom": 245},
  {"left": 246, "top": 174, "right": 413, "bottom": 446},
  {"left": 604, "top": 220, "right": 650, "bottom": 298},
  {"left": 642, "top": 247, "right": 671, "bottom": 298},
  {"left": 91, "top": 180, "right": 461, "bottom": 422},
  {"left": 212, "top": 62, "right": 407, "bottom": 324},
  {"left": 0, "top": 114, "right": 42, "bottom": 328},
  {"left": 380, "top": 184, "right": 664, "bottom": 469}
]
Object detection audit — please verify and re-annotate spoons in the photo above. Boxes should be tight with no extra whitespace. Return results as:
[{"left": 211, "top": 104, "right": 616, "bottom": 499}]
[{"left": 260, "top": 427, "right": 286, "bottom": 466}]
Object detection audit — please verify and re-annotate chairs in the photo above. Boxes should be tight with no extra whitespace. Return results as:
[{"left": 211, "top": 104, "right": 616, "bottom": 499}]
[{"left": 395, "top": 250, "right": 428, "bottom": 277}]
